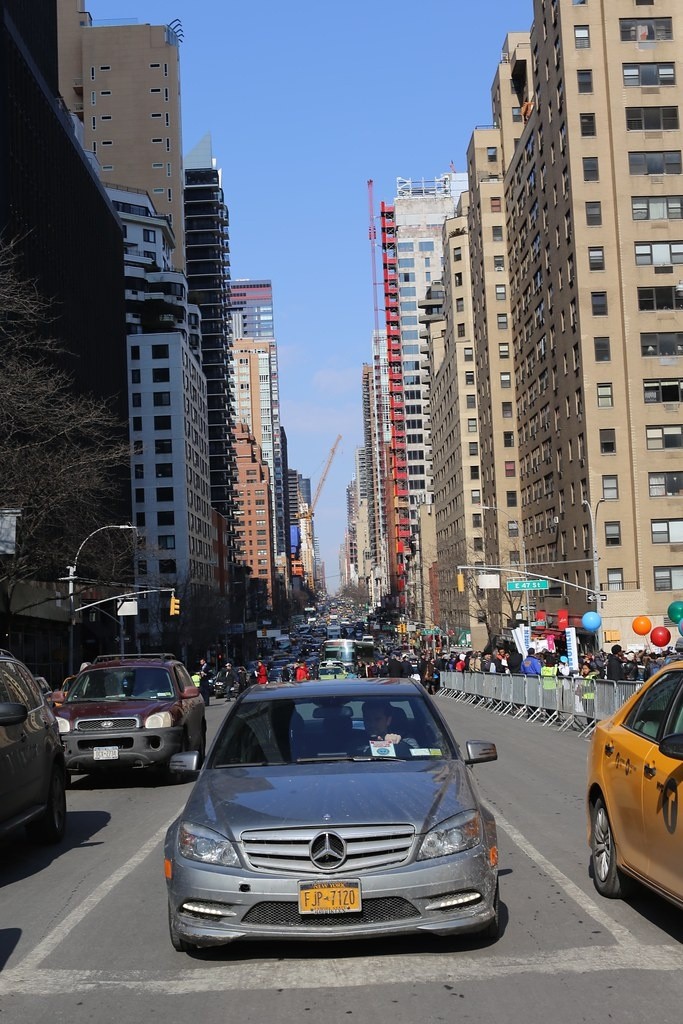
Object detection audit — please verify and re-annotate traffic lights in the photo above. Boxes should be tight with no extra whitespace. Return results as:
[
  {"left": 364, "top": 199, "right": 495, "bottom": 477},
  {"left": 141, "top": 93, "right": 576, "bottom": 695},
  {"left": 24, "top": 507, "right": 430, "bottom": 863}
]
[
  {"left": 397, "top": 625, "right": 400, "bottom": 633},
  {"left": 175, "top": 599, "right": 180, "bottom": 615},
  {"left": 405, "top": 624, "right": 407, "bottom": 633}
]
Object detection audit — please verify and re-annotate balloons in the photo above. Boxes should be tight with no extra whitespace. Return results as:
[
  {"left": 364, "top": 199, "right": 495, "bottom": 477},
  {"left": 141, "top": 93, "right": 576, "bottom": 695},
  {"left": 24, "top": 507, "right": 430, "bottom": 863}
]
[
  {"left": 668, "top": 601, "right": 683, "bottom": 623},
  {"left": 582, "top": 612, "right": 601, "bottom": 632},
  {"left": 632, "top": 616, "right": 652, "bottom": 636},
  {"left": 650, "top": 626, "right": 671, "bottom": 647},
  {"left": 678, "top": 618, "right": 683, "bottom": 636}
]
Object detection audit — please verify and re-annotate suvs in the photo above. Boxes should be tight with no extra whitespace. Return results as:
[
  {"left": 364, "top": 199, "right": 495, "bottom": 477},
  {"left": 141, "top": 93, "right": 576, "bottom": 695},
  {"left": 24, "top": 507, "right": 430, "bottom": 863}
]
[{"left": 54, "top": 654, "right": 208, "bottom": 786}]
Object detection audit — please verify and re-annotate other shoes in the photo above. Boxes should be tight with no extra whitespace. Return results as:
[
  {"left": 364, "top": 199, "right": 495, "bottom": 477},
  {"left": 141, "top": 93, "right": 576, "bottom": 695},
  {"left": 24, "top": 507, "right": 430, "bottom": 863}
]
[
  {"left": 235, "top": 694, "right": 240, "bottom": 700},
  {"left": 225, "top": 699, "right": 231, "bottom": 702}
]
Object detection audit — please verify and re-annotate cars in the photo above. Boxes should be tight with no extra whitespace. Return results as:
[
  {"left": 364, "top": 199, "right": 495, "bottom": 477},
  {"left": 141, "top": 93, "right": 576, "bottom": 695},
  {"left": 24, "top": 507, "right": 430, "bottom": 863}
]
[
  {"left": 584, "top": 660, "right": 683, "bottom": 909},
  {"left": 33, "top": 673, "right": 77, "bottom": 708},
  {"left": 0, "top": 649, "right": 69, "bottom": 850},
  {"left": 248, "top": 597, "right": 420, "bottom": 685},
  {"left": 164, "top": 681, "right": 501, "bottom": 952}
]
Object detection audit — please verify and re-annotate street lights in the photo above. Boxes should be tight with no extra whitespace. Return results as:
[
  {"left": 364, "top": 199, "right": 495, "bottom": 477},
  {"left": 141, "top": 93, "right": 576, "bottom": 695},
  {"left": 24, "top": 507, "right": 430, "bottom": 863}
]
[
  {"left": 582, "top": 498, "right": 608, "bottom": 651},
  {"left": 68, "top": 524, "right": 137, "bottom": 677}
]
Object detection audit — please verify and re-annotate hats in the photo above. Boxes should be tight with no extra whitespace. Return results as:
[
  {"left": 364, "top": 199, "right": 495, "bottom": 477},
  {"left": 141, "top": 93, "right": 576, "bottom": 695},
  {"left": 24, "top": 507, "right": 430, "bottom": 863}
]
[
  {"left": 540, "top": 648, "right": 548, "bottom": 655},
  {"left": 225, "top": 663, "right": 232, "bottom": 666}
]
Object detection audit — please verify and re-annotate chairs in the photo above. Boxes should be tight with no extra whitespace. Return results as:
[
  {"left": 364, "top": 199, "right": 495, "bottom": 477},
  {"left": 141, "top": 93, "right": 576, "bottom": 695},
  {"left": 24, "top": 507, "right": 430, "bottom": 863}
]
[{"left": 387, "top": 708, "right": 412, "bottom": 744}]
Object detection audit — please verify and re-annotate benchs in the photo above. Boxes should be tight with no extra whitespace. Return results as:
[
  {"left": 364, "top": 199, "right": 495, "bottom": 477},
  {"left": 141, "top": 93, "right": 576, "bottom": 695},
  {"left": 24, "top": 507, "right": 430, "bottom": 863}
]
[{"left": 300, "top": 718, "right": 368, "bottom": 755}]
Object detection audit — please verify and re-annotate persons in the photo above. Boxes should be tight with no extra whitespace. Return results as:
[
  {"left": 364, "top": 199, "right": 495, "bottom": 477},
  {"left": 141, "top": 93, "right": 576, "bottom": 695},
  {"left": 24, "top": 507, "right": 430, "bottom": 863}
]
[
  {"left": 435, "top": 645, "right": 672, "bottom": 728},
  {"left": 199, "top": 659, "right": 214, "bottom": 707},
  {"left": 225, "top": 662, "right": 234, "bottom": 702},
  {"left": 427, "top": 657, "right": 437, "bottom": 695},
  {"left": 238, "top": 666, "right": 248, "bottom": 694},
  {"left": 371, "top": 655, "right": 415, "bottom": 678},
  {"left": 362, "top": 701, "right": 419, "bottom": 749},
  {"left": 418, "top": 653, "right": 427, "bottom": 688},
  {"left": 280, "top": 665, "right": 290, "bottom": 682},
  {"left": 256, "top": 660, "right": 267, "bottom": 685},
  {"left": 293, "top": 660, "right": 310, "bottom": 681},
  {"left": 355, "top": 660, "right": 366, "bottom": 678}
]
